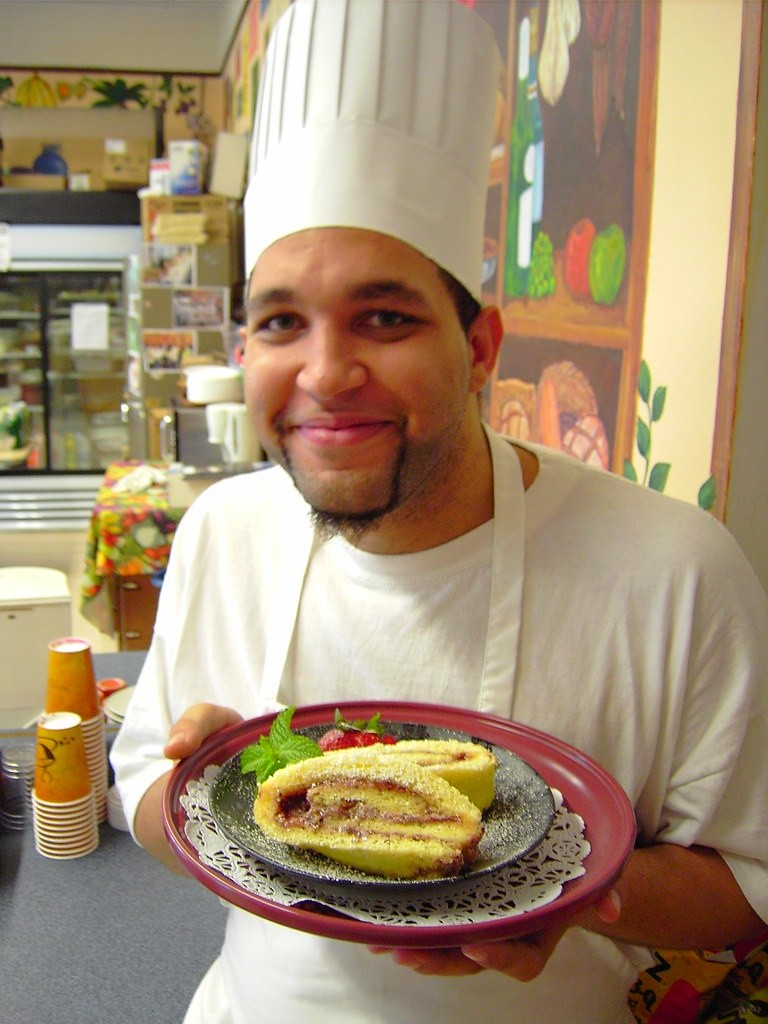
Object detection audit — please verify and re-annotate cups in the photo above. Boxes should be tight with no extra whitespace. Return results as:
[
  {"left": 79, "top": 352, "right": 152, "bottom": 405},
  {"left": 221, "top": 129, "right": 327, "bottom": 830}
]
[{"left": 33, "top": 637, "right": 109, "bottom": 860}]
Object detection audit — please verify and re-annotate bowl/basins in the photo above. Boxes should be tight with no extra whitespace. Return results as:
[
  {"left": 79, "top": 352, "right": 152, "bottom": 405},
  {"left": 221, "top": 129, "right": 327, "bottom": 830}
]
[{"left": 186, "top": 367, "right": 241, "bottom": 403}]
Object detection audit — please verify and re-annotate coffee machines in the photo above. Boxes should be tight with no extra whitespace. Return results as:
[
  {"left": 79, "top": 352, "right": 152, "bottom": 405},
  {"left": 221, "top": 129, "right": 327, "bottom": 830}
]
[{"left": 206, "top": 405, "right": 261, "bottom": 468}]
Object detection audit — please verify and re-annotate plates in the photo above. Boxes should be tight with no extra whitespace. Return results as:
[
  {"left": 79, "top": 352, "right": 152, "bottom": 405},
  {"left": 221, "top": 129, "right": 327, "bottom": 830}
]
[
  {"left": 208, "top": 721, "right": 557, "bottom": 888},
  {"left": 162, "top": 697, "right": 637, "bottom": 949}
]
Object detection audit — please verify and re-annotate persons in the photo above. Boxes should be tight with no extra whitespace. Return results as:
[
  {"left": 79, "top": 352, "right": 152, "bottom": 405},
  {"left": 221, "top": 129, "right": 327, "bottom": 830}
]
[{"left": 111, "top": 0, "right": 768, "bottom": 1024}]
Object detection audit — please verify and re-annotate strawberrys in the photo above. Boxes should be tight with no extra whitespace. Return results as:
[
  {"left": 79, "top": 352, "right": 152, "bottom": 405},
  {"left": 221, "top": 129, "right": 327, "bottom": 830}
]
[{"left": 317, "top": 727, "right": 398, "bottom": 751}]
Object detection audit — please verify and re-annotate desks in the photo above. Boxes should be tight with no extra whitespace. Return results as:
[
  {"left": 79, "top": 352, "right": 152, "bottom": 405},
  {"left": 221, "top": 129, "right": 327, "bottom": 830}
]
[{"left": 80, "top": 458, "right": 202, "bottom": 652}]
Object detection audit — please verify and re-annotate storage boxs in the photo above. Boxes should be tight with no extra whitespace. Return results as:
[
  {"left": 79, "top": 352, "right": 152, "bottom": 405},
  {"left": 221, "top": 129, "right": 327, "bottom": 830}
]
[{"left": 139, "top": 192, "right": 233, "bottom": 245}]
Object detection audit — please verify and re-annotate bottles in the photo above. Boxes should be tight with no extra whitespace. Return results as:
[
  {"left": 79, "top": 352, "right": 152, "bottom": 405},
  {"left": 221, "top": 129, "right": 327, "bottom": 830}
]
[{"left": 34, "top": 145, "right": 66, "bottom": 176}]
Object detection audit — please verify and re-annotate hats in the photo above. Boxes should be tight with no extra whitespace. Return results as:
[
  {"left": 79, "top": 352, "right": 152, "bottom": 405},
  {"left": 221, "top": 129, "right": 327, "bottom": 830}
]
[{"left": 243, "top": 0, "right": 500, "bottom": 310}]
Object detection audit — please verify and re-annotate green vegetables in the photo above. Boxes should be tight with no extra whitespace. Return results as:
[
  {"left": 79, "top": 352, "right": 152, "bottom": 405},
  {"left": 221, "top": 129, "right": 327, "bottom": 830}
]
[{"left": 240, "top": 705, "right": 324, "bottom": 787}]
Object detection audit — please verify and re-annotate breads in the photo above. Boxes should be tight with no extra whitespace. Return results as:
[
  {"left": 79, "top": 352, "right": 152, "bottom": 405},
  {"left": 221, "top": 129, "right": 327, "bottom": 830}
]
[
  {"left": 315, "top": 738, "right": 497, "bottom": 813},
  {"left": 253, "top": 751, "right": 486, "bottom": 881}
]
